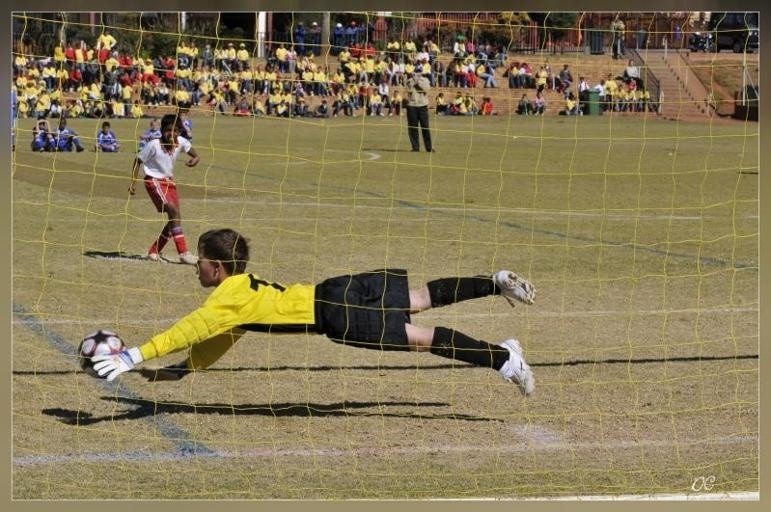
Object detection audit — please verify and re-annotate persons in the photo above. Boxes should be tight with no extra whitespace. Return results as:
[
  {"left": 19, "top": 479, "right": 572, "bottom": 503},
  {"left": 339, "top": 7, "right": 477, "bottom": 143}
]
[
  {"left": 127, "top": 115, "right": 199, "bottom": 264},
  {"left": 90, "top": 228, "right": 536, "bottom": 396},
  {"left": 10, "top": 15, "right": 684, "bottom": 154}
]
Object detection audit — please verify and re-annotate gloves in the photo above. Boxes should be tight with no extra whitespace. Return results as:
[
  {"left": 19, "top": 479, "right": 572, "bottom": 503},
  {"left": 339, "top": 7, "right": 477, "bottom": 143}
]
[
  {"left": 91, "top": 347, "right": 144, "bottom": 382},
  {"left": 140, "top": 362, "right": 190, "bottom": 381}
]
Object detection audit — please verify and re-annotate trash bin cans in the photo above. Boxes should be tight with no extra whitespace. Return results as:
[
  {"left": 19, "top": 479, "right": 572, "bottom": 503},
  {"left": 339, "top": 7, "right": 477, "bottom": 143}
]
[{"left": 583, "top": 89, "right": 600, "bottom": 115}]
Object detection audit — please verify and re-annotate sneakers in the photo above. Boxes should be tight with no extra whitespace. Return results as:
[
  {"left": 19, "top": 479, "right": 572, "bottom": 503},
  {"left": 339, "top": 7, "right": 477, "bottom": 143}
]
[
  {"left": 181, "top": 254, "right": 200, "bottom": 264},
  {"left": 499, "top": 338, "right": 536, "bottom": 396},
  {"left": 493, "top": 270, "right": 537, "bottom": 306},
  {"left": 149, "top": 253, "right": 169, "bottom": 263}
]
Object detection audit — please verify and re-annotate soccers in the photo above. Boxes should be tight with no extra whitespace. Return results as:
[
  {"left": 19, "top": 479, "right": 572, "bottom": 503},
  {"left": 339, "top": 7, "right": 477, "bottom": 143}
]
[{"left": 78, "top": 329, "right": 126, "bottom": 378}]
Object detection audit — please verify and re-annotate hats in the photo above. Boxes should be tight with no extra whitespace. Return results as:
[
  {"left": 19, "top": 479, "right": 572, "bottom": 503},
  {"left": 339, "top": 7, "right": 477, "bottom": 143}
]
[{"left": 415, "top": 64, "right": 424, "bottom": 71}]
